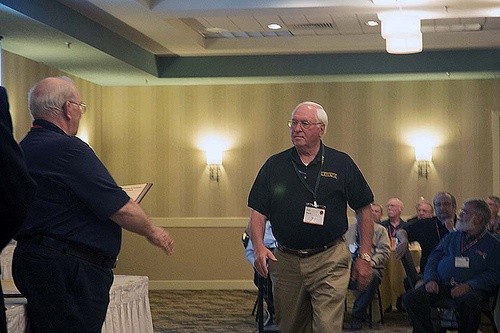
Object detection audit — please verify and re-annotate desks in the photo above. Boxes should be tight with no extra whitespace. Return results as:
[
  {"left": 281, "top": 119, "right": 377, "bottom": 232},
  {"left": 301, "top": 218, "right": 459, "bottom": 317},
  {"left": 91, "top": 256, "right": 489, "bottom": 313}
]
[{"left": 99, "top": 274, "right": 154, "bottom": 333}]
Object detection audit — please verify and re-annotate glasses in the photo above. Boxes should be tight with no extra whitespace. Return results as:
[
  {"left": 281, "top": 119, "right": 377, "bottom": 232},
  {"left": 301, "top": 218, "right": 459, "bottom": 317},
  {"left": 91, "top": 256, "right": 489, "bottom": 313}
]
[
  {"left": 287, "top": 119, "right": 323, "bottom": 129},
  {"left": 68, "top": 100, "right": 88, "bottom": 114},
  {"left": 459, "top": 208, "right": 469, "bottom": 215}
]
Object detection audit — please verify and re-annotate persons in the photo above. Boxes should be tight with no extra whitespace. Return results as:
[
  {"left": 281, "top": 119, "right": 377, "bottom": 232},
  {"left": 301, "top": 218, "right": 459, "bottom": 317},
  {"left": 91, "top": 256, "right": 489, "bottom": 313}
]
[
  {"left": 343, "top": 211, "right": 390, "bottom": 331},
  {"left": 245, "top": 221, "right": 280, "bottom": 315},
  {"left": 379, "top": 197, "right": 411, "bottom": 312},
  {"left": 248, "top": 101, "right": 375, "bottom": 333},
  {"left": 370, "top": 203, "right": 383, "bottom": 224},
  {"left": 486, "top": 196, "right": 500, "bottom": 242},
  {"left": 402, "top": 201, "right": 500, "bottom": 333},
  {"left": 417, "top": 202, "right": 434, "bottom": 219},
  {"left": 0, "top": 84, "right": 37, "bottom": 333},
  {"left": 10, "top": 76, "right": 175, "bottom": 333},
  {"left": 394, "top": 191, "right": 459, "bottom": 282},
  {"left": 407, "top": 196, "right": 429, "bottom": 223}
]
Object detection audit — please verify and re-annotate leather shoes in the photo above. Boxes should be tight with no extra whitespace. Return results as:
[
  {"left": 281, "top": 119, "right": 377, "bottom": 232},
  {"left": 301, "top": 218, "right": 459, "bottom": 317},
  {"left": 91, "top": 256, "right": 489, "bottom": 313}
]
[{"left": 351, "top": 318, "right": 361, "bottom": 331}]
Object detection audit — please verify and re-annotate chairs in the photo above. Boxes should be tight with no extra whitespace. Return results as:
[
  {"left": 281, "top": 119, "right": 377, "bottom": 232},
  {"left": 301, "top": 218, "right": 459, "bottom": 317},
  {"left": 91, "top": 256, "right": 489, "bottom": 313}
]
[{"left": 343, "top": 269, "right": 384, "bottom": 329}]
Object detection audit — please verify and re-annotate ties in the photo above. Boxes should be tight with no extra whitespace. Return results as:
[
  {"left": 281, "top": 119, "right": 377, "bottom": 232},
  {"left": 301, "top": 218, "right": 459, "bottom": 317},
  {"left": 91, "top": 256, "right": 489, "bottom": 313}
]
[{"left": 392, "top": 228, "right": 397, "bottom": 237}]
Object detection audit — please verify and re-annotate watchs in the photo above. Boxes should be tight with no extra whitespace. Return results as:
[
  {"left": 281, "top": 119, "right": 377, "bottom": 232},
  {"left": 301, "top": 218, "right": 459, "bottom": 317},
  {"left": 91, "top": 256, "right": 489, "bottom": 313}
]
[{"left": 356, "top": 251, "right": 372, "bottom": 261}]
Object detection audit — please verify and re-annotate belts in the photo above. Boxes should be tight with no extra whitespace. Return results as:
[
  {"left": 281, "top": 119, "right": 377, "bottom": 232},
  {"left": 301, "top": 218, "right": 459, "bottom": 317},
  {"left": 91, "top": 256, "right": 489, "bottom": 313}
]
[
  {"left": 26, "top": 235, "right": 118, "bottom": 269},
  {"left": 277, "top": 237, "right": 345, "bottom": 258}
]
